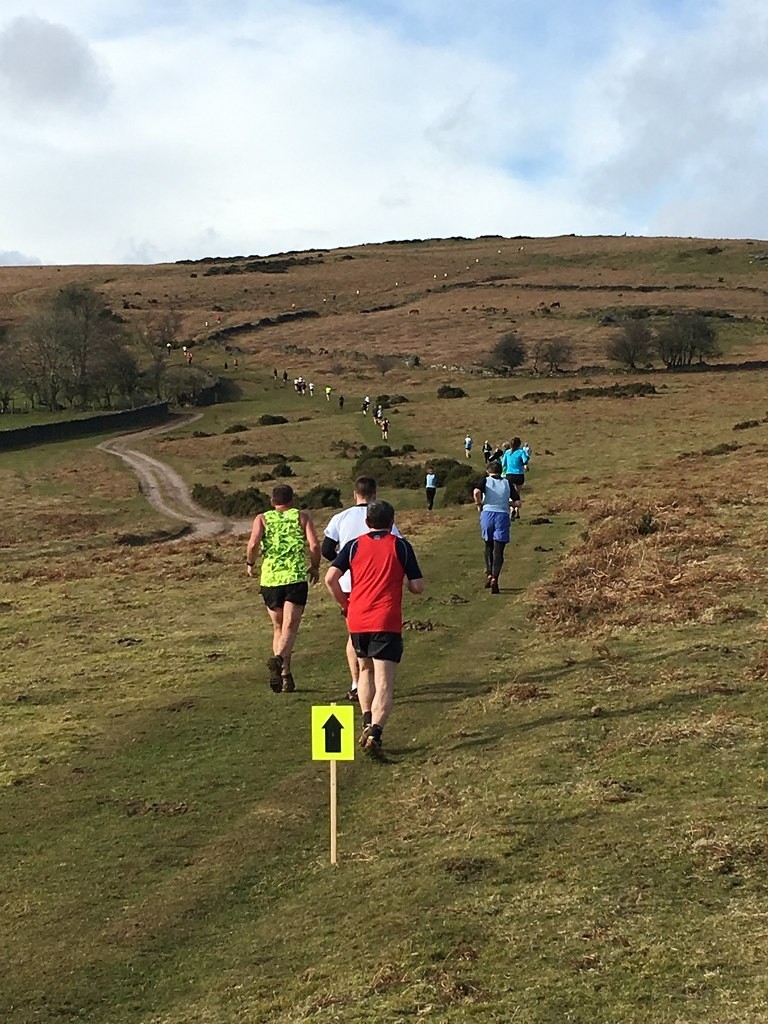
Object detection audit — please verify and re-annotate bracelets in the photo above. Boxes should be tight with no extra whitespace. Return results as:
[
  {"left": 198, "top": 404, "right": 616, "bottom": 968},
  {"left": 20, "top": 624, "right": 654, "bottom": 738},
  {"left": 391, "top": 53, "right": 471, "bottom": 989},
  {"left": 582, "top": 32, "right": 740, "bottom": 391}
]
[{"left": 246, "top": 561, "right": 255, "bottom": 566}]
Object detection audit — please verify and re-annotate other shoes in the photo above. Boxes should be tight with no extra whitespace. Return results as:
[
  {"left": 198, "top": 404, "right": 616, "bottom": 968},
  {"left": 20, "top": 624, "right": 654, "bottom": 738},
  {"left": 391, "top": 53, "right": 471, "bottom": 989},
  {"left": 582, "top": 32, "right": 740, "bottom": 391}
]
[
  {"left": 485, "top": 575, "right": 491, "bottom": 588},
  {"left": 511, "top": 510, "right": 516, "bottom": 522},
  {"left": 515, "top": 514, "right": 520, "bottom": 518},
  {"left": 428, "top": 503, "right": 432, "bottom": 510},
  {"left": 490, "top": 578, "right": 499, "bottom": 594}
]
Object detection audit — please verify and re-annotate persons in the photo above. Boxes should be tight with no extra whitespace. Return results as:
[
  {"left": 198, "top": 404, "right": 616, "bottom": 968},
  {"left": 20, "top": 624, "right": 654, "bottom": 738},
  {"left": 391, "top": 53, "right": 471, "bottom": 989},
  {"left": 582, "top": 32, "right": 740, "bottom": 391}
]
[
  {"left": 246, "top": 484, "right": 320, "bottom": 693},
  {"left": 482, "top": 440, "right": 532, "bottom": 473},
  {"left": 325, "top": 500, "right": 425, "bottom": 751},
  {"left": 457, "top": 258, "right": 479, "bottom": 274},
  {"left": 323, "top": 298, "right": 327, "bottom": 305},
  {"left": 273, "top": 368, "right": 344, "bottom": 409},
  {"left": 517, "top": 245, "right": 524, "bottom": 253},
  {"left": 425, "top": 467, "right": 439, "bottom": 510},
  {"left": 463, "top": 434, "right": 473, "bottom": 458},
  {"left": 501, "top": 437, "right": 530, "bottom": 521},
  {"left": 224, "top": 359, "right": 239, "bottom": 373},
  {"left": 333, "top": 294, "right": 336, "bottom": 301},
  {"left": 433, "top": 272, "right": 448, "bottom": 280},
  {"left": 166, "top": 342, "right": 193, "bottom": 365},
  {"left": 473, "top": 461, "right": 522, "bottom": 594},
  {"left": 361, "top": 395, "right": 391, "bottom": 442},
  {"left": 356, "top": 289, "right": 360, "bottom": 297},
  {"left": 321, "top": 475, "right": 403, "bottom": 701},
  {"left": 291, "top": 301, "right": 297, "bottom": 311},
  {"left": 204, "top": 315, "right": 221, "bottom": 330},
  {"left": 395, "top": 280, "right": 398, "bottom": 287},
  {"left": 497, "top": 249, "right": 502, "bottom": 254}
]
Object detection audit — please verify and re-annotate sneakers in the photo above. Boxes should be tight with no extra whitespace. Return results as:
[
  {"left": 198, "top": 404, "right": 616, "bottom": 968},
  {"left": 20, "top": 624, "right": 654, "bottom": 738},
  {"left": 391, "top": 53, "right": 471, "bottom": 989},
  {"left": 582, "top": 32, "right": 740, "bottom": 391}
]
[
  {"left": 282, "top": 678, "right": 294, "bottom": 692},
  {"left": 363, "top": 735, "right": 387, "bottom": 764},
  {"left": 359, "top": 721, "right": 368, "bottom": 748},
  {"left": 267, "top": 655, "right": 283, "bottom": 693},
  {"left": 345, "top": 688, "right": 358, "bottom": 702}
]
